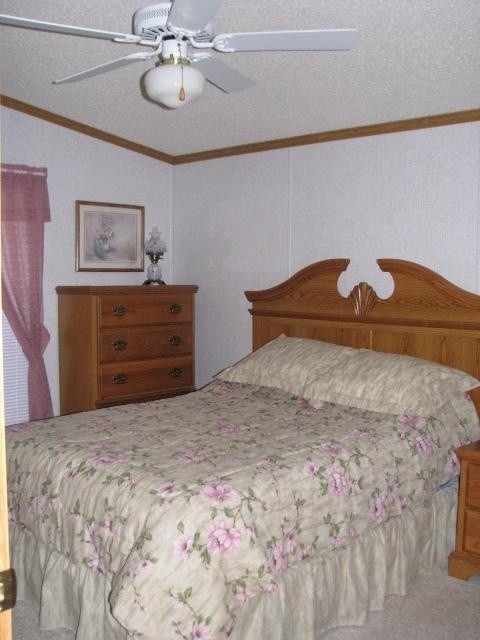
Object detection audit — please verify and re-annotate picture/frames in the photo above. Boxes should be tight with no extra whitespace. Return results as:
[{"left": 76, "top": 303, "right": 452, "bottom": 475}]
[{"left": 75, "top": 200, "right": 146, "bottom": 273}]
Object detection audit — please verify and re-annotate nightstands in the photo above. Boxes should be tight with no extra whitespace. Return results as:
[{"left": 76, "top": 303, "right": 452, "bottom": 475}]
[{"left": 447, "top": 438, "right": 480, "bottom": 582}]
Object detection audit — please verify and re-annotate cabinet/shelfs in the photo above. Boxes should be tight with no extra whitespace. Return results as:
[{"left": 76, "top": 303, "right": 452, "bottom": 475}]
[{"left": 55, "top": 285, "right": 199, "bottom": 417}]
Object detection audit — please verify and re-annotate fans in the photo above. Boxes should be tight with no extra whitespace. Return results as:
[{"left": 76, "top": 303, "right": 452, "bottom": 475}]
[{"left": 0, "top": 0, "right": 360, "bottom": 95}]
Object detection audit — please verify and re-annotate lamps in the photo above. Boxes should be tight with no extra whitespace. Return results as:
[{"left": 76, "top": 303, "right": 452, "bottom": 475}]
[
  {"left": 144, "top": 39, "right": 205, "bottom": 110},
  {"left": 142, "top": 226, "right": 168, "bottom": 286}
]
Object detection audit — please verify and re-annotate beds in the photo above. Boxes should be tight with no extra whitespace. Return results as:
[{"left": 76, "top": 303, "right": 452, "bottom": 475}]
[{"left": 5, "top": 256, "right": 480, "bottom": 640}]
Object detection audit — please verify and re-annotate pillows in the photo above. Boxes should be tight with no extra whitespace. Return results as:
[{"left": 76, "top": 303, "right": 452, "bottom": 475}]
[
  {"left": 302, "top": 348, "right": 480, "bottom": 417},
  {"left": 212, "top": 333, "right": 372, "bottom": 410}
]
